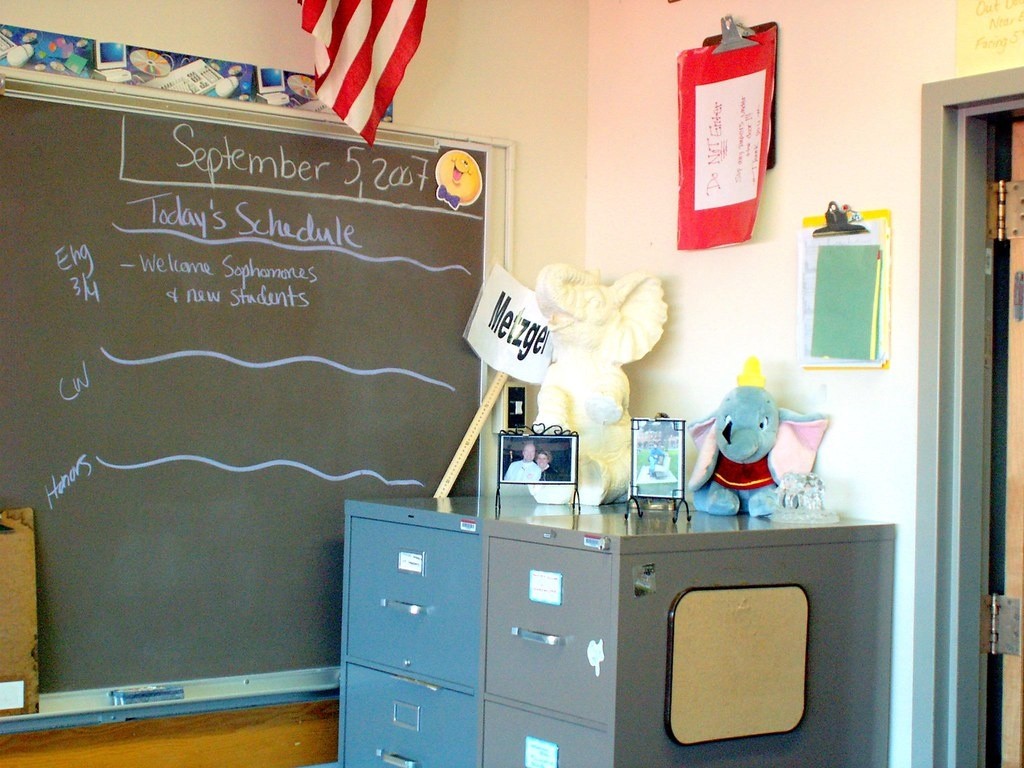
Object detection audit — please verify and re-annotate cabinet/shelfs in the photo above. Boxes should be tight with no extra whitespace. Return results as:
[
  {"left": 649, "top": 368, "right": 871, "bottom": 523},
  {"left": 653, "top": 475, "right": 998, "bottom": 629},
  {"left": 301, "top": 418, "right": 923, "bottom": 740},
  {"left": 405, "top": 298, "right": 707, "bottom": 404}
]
[{"left": 336, "top": 498, "right": 898, "bottom": 768}]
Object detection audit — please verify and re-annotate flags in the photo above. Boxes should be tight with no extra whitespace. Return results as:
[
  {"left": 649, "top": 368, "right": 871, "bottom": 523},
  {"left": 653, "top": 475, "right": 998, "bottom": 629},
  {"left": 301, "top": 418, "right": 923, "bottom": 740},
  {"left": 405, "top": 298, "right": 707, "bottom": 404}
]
[{"left": 297, "top": 0, "right": 428, "bottom": 146}]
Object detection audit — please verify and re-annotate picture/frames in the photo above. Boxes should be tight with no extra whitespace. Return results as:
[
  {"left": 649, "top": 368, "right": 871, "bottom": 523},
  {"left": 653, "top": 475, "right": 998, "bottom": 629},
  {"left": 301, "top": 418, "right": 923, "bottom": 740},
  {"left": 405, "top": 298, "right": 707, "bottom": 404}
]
[
  {"left": 629, "top": 417, "right": 687, "bottom": 499},
  {"left": 497, "top": 433, "right": 579, "bottom": 486}
]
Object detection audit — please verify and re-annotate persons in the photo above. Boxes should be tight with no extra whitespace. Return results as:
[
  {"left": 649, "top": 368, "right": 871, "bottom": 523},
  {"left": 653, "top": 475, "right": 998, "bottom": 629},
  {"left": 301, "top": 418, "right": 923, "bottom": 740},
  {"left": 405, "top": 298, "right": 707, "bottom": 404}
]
[
  {"left": 537, "top": 450, "right": 561, "bottom": 481},
  {"left": 649, "top": 443, "right": 666, "bottom": 476},
  {"left": 503, "top": 444, "right": 540, "bottom": 481}
]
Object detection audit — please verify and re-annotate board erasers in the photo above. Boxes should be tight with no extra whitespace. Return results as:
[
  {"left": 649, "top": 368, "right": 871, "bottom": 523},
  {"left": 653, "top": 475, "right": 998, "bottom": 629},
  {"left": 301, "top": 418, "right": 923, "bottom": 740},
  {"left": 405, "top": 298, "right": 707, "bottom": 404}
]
[{"left": 107, "top": 685, "right": 185, "bottom": 707}]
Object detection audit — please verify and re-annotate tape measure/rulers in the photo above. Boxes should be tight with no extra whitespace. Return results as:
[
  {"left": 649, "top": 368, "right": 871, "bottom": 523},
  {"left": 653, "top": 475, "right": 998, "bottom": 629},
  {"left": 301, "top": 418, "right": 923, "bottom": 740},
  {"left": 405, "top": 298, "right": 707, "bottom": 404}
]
[{"left": 433, "top": 370, "right": 509, "bottom": 499}]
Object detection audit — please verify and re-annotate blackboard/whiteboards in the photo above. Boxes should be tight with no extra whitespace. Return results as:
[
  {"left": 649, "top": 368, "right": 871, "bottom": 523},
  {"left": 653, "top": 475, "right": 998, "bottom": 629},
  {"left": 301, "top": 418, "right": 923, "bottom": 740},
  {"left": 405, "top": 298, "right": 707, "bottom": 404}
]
[{"left": 0, "top": 65, "right": 518, "bottom": 738}]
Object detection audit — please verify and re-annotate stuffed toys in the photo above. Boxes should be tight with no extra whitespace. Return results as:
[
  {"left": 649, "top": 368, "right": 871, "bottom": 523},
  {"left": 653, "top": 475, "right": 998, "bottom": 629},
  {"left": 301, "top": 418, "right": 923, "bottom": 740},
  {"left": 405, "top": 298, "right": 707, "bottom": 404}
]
[
  {"left": 526, "top": 264, "right": 668, "bottom": 505},
  {"left": 688, "top": 357, "right": 829, "bottom": 517}
]
